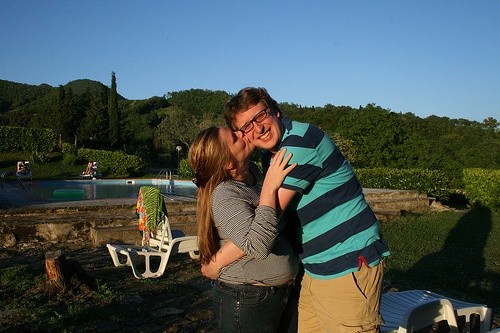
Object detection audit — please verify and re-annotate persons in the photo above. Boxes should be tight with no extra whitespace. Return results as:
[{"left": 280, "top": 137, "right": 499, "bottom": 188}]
[
  {"left": 85, "top": 162, "right": 97, "bottom": 175},
  {"left": 201, "top": 87, "right": 390, "bottom": 333},
  {"left": 17, "top": 160, "right": 30, "bottom": 174},
  {"left": 188, "top": 127, "right": 304, "bottom": 333}
]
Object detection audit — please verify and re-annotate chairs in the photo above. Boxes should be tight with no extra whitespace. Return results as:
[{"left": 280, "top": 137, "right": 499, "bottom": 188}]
[
  {"left": 106, "top": 186, "right": 203, "bottom": 279},
  {"left": 378, "top": 289, "right": 492, "bottom": 333}
]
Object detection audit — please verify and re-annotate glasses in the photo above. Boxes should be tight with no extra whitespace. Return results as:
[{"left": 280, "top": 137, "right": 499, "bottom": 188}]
[{"left": 239, "top": 106, "right": 271, "bottom": 134}]
